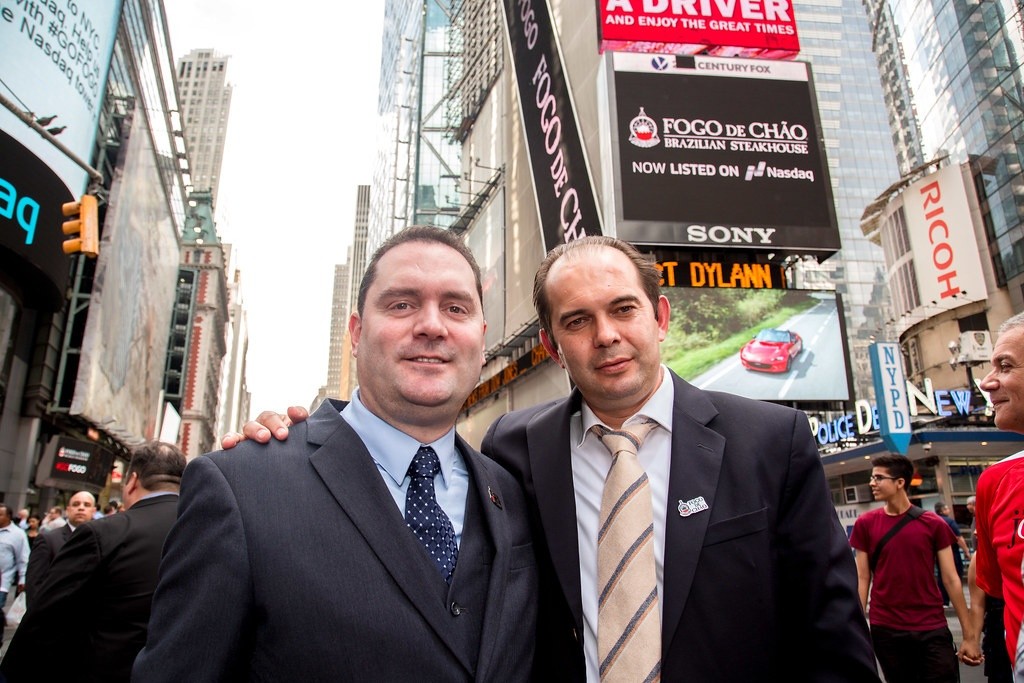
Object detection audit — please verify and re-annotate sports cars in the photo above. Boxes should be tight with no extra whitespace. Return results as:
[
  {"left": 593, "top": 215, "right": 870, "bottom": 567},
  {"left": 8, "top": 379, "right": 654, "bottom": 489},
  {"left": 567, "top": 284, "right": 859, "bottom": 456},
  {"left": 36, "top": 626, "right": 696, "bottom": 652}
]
[{"left": 740, "top": 328, "right": 803, "bottom": 373}]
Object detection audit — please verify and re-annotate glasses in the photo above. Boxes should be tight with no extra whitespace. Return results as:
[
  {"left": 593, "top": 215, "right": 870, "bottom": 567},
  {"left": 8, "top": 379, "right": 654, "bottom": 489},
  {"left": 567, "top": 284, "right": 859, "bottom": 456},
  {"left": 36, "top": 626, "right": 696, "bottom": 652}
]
[{"left": 870, "top": 475, "right": 895, "bottom": 481}]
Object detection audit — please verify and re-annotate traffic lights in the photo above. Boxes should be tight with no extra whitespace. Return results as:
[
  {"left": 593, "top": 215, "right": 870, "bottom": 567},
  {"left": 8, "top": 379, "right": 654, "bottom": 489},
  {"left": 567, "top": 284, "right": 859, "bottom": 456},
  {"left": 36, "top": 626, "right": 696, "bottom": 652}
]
[{"left": 62, "top": 194, "right": 99, "bottom": 259}]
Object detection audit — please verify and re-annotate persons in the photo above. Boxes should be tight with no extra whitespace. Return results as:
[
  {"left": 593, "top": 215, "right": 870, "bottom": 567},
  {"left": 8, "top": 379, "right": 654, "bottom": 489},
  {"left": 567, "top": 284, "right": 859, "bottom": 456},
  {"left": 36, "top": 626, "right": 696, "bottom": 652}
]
[
  {"left": 935, "top": 503, "right": 970, "bottom": 609},
  {"left": 850, "top": 453, "right": 981, "bottom": 683},
  {"left": 130, "top": 226, "right": 539, "bottom": 683},
  {"left": 0, "top": 442, "right": 187, "bottom": 683},
  {"left": 955, "top": 530, "right": 1013, "bottom": 683},
  {"left": 976, "top": 313, "right": 1024, "bottom": 683},
  {"left": 0, "top": 491, "right": 126, "bottom": 649},
  {"left": 221, "top": 235, "right": 879, "bottom": 683},
  {"left": 967, "top": 495, "right": 977, "bottom": 552}
]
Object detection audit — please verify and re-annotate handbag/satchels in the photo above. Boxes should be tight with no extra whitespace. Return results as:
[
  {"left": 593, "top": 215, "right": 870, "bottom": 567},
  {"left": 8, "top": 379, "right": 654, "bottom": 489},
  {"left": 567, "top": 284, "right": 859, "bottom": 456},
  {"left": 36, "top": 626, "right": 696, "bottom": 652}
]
[{"left": 6, "top": 591, "right": 29, "bottom": 624}]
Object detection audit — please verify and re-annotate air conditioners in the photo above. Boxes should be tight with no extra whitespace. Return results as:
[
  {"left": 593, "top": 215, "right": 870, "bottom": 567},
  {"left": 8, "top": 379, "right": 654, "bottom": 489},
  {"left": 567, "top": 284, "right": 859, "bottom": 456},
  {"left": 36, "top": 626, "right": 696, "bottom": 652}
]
[{"left": 843, "top": 483, "right": 873, "bottom": 505}]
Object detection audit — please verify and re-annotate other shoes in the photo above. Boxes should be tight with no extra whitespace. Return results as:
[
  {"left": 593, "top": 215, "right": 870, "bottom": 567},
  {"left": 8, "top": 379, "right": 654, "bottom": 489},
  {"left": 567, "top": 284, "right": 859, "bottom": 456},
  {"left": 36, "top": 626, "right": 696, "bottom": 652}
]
[{"left": 943, "top": 602, "right": 953, "bottom": 608}]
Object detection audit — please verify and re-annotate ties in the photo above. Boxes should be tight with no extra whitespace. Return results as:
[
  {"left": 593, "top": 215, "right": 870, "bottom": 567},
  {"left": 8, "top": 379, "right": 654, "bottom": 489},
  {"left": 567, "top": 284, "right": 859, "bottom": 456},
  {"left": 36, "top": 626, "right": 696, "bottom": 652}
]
[
  {"left": 591, "top": 418, "right": 659, "bottom": 683},
  {"left": 404, "top": 447, "right": 458, "bottom": 586}
]
